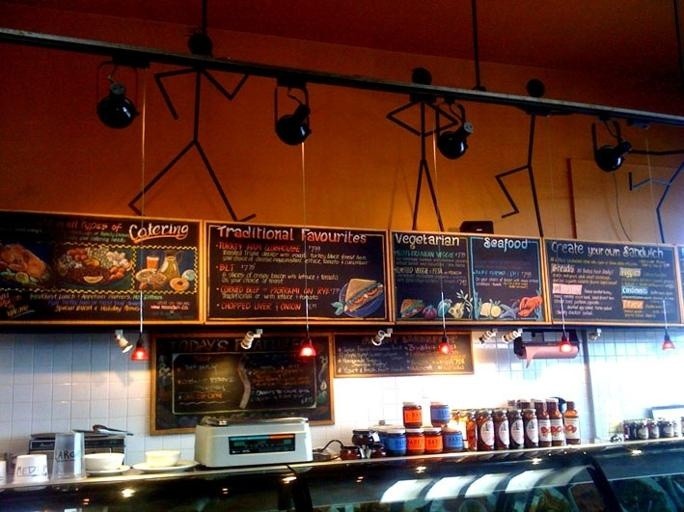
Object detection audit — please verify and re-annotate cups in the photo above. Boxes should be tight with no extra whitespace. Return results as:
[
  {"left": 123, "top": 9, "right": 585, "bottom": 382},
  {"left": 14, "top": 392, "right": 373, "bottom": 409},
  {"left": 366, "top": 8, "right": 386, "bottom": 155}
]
[
  {"left": 0, "top": 460, "right": 6, "bottom": 485},
  {"left": 146, "top": 255, "right": 159, "bottom": 271},
  {"left": 50, "top": 431, "right": 85, "bottom": 481},
  {"left": 12, "top": 454, "right": 48, "bottom": 486}
]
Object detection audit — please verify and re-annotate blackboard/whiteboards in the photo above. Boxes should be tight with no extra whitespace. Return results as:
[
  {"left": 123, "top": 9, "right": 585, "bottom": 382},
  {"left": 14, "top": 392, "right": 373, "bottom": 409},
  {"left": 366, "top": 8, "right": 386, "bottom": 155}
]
[
  {"left": 0, "top": 208, "right": 203, "bottom": 324},
  {"left": 149, "top": 331, "right": 334, "bottom": 435},
  {"left": 333, "top": 332, "right": 473, "bottom": 375},
  {"left": 203, "top": 221, "right": 391, "bottom": 326},
  {"left": 542, "top": 239, "right": 684, "bottom": 328},
  {"left": 384, "top": 228, "right": 547, "bottom": 323}
]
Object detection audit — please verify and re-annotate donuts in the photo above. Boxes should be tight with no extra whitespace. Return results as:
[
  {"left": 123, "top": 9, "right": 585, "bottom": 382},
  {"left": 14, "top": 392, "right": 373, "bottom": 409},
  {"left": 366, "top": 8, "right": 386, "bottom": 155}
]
[{"left": 171, "top": 277, "right": 190, "bottom": 292}]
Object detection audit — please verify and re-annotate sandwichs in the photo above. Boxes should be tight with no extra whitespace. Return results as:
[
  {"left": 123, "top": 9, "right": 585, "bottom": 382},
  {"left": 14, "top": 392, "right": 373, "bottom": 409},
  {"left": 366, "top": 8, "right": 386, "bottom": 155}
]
[
  {"left": 400, "top": 298, "right": 424, "bottom": 317},
  {"left": 344, "top": 278, "right": 383, "bottom": 313}
]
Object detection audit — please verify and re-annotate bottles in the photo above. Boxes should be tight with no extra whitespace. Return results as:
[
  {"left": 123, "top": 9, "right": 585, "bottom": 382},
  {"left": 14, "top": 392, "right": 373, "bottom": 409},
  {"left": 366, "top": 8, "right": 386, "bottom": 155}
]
[
  {"left": 156, "top": 252, "right": 180, "bottom": 284},
  {"left": 615, "top": 416, "right": 684, "bottom": 440},
  {"left": 451, "top": 397, "right": 581, "bottom": 452}
]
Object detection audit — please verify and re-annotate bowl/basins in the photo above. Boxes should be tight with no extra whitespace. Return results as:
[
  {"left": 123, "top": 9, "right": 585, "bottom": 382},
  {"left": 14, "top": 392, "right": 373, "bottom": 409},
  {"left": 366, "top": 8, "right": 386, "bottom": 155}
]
[
  {"left": 144, "top": 450, "right": 181, "bottom": 468},
  {"left": 82, "top": 452, "right": 124, "bottom": 469}
]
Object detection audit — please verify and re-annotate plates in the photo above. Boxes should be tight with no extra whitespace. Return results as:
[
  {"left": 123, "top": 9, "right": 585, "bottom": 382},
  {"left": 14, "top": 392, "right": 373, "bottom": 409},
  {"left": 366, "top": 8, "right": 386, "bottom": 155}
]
[
  {"left": 338, "top": 283, "right": 383, "bottom": 318},
  {"left": 84, "top": 464, "right": 130, "bottom": 474},
  {"left": 132, "top": 459, "right": 198, "bottom": 474}
]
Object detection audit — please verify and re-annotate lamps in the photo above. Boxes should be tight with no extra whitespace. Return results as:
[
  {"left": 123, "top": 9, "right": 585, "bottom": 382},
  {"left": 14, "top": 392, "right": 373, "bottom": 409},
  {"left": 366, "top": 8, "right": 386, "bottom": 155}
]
[
  {"left": 130, "top": 48, "right": 151, "bottom": 363},
  {"left": 271, "top": 77, "right": 317, "bottom": 145},
  {"left": 435, "top": 95, "right": 475, "bottom": 160},
  {"left": 475, "top": 328, "right": 498, "bottom": 345},
  {"left": 92, "top": 53, "right": 141, "bottom": 129},
  {"left": 240, "top": 328, "right": 264, "bottom": 350},
  {"left": 592, "top": 117, "right": 632, "bottom": 175},
  {"left": 637, "top": 118, "right": 676, "bottom": 352},
  {"left": 589, "top": 327, "right": 603, "bottom": 343},
  {"left": 501, "top": 326, "right": 523, "bottom": 345},
  {"left": 114, "top": 328, "right": 133, "bottom": 354},
  {"left": 371, "top": 326, "right": 393, "bottom": 347},
  {"left": 431, "top": 101, "right": 455, "bottom": 356},
  {"left": 294, "top": 138, "right": 318, "bottom": 360},
  {"left": 543, "top": 103, "right": 574, "bottom": 354}
]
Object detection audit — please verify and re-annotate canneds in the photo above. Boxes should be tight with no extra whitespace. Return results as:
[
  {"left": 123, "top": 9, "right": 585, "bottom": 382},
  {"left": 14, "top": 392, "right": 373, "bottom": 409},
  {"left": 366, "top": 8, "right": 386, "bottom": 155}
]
[
  {"left": 340, "top": 401, "right": 540, "bottom": 460},
  {"left": 624, "top": 416, "right": 684, "bottom": 440}
]
